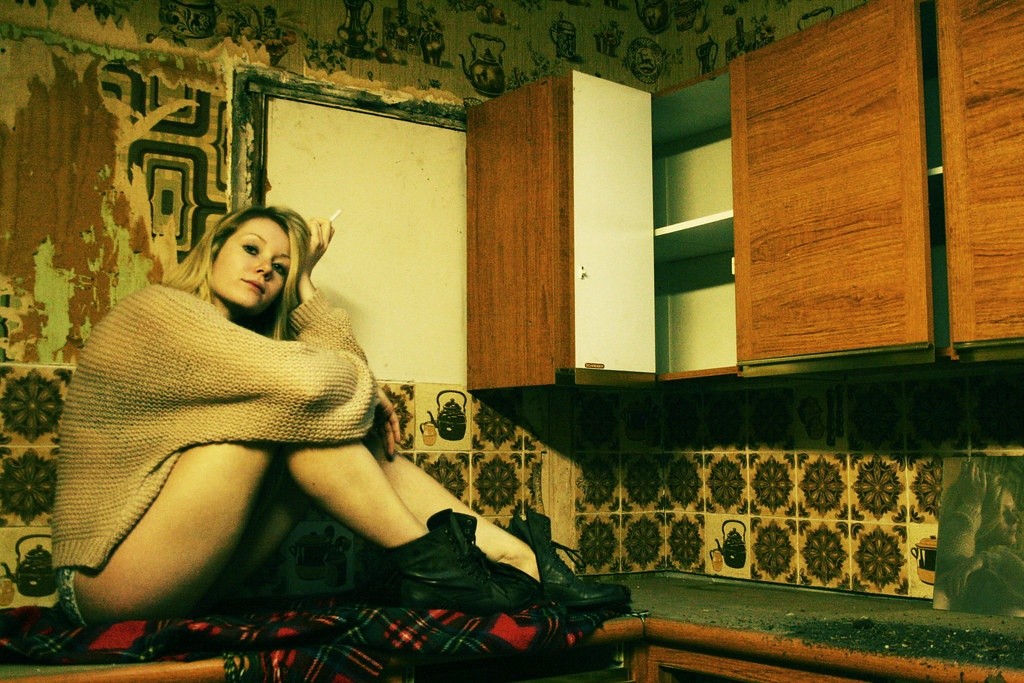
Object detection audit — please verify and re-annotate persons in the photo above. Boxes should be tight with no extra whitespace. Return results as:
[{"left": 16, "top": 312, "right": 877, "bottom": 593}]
[
  {"left": 51, "top": 206, "right": 630, "bottom": 625},
  {"left": 935, "top": 460, "right": 1024, "bottom": 596}
]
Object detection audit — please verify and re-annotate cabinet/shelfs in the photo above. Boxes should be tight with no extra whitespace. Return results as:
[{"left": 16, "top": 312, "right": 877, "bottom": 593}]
[
  {"left": 464, "top": 66, "right": 660, "bottom": 393},
  {"left": 653, "top": 2, "right": 1023, "bottom": 384}
]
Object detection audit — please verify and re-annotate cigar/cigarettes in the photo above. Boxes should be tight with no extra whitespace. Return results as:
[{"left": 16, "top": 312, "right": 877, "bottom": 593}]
[{"left": 329, "top": 210, "right": 342, "bottom": 221}]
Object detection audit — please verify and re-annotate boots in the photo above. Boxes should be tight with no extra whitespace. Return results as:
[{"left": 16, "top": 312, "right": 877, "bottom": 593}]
[
  {"left": 378, "top": 510, "right": 534, "bottom": 616},
  {"left": 504, "top": 509, "right": 632, "bottom": 616}
]
